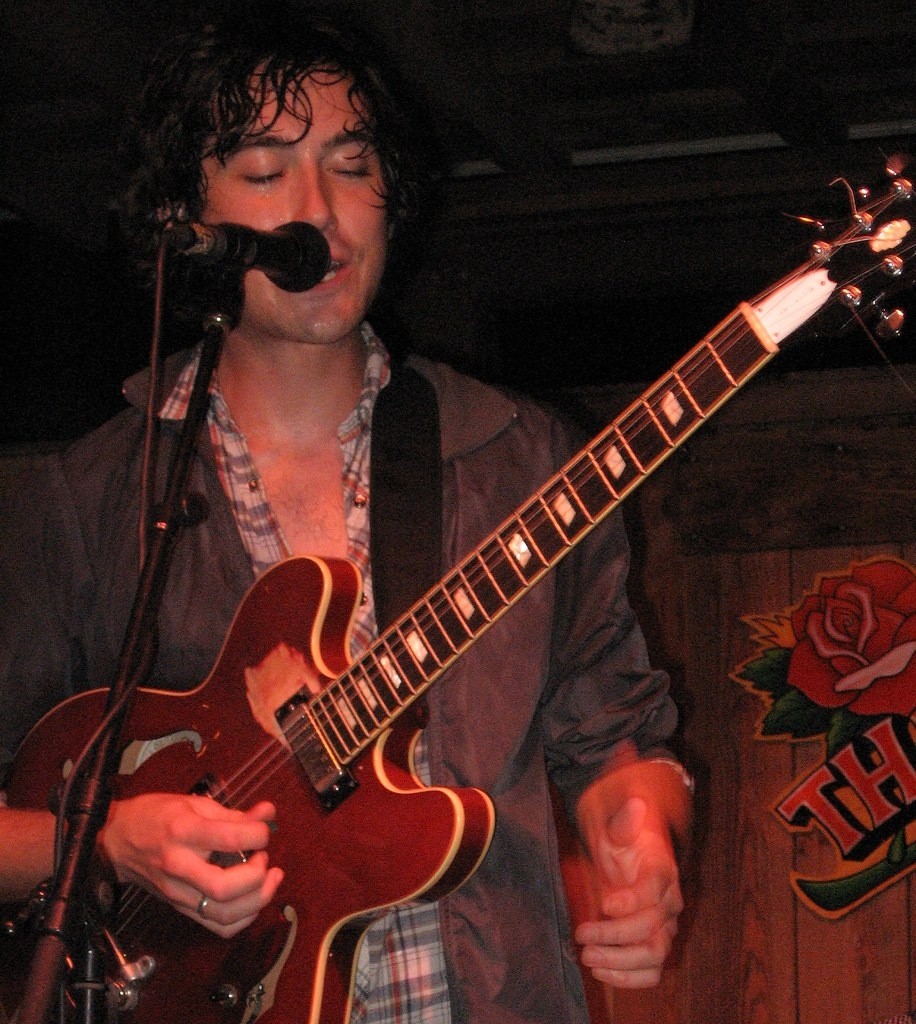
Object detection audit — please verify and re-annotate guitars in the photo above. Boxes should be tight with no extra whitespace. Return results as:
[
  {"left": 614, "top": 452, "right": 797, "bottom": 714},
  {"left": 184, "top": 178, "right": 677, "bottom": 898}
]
[{"left": 0, "top": 148, "right": 916, "bottom": 1024}]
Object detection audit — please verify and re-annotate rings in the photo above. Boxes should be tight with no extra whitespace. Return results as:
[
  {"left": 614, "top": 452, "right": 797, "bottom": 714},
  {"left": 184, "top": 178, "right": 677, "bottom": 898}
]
[{"left": 197, "top": 895, "right": 210, "bottom": 919}]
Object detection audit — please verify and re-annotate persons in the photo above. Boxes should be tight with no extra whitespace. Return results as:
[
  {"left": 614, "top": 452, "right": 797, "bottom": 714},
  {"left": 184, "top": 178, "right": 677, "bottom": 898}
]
[{"left": 0, "top": 25, "right": 690, "bottom": 1024}]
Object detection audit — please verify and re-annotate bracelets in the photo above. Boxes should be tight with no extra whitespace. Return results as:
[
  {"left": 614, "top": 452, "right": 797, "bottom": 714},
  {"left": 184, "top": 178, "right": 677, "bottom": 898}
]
[{"left": 646, "top": 758, "right": 695, "bottom": 790}]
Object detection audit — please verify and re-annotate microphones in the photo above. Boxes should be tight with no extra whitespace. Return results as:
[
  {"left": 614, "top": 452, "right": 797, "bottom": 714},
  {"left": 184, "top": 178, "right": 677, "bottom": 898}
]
[{"left": 170, "top": 220, "right": 330, "bottom": 293}]
